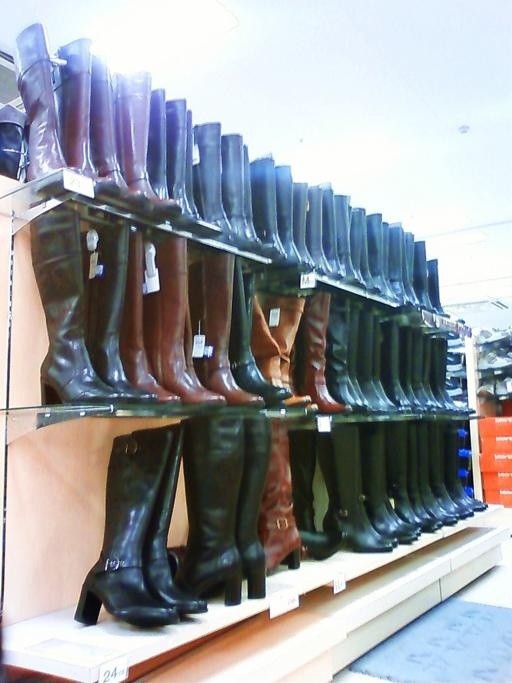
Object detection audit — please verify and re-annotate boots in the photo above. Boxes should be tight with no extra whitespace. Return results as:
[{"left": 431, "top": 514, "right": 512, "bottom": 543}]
[
  {"left": 12, "top": 21, "right": 82, "bottom": 181},
  {"left": 92, "top": 56, "right": 149, "bottom": 212},
  {"left": 217, "top": 406, "right": 266, "bottom": 599},
  {"left": 131, "top": 422, "right": 208, "bottom": 615},
  {"left": 180, "top": 413, "right": 242, "bottom": 605},
  {"left": 81, "top": 222, "right": 158, "bottom": 402},
  {"left": 252, "top": 284, "right": 476, "bottom": 414},
  {"left": 74, "top": 429, "right": 179, "bottom": 628},
  {"left": 51, "top": 37, "right": 121, "bottom": 204},
  {"left": 120, "top": 230, "right": 182, "bottom": 403},
  {"left": 258, "top": 415, "right": 301, "bottom": 573},
  {"left": 116, "top": 68, "right": 183, "bottom": 222},
  {"left": 188, "top": 251, "right": 266, "bottom": 408},
  {"left": 287, "top": 428, "right": 317, "bottom": 549},
  {"left": 144, "top": 236, "right": 227, "bottom": 405},
  {"left": 228, "top": 254, "right": 292, "bottom": 406},
  {"left": 29, "top": 199, "right": 121, "bottom": 403},
  {"left": 321, "top": 422, "right": 487, "bottom": 551}
]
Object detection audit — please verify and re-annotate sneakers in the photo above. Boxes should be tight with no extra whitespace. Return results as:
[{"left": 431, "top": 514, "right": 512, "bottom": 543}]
[
  {"left": 478, "top": 349, "right": 511, "bottom": 369},
  {"left": 473, "top": 325, "right": 511, "bottom": 345},
  {"left": 476, "top": 377, "right": 494, "bottom": 397}
]
